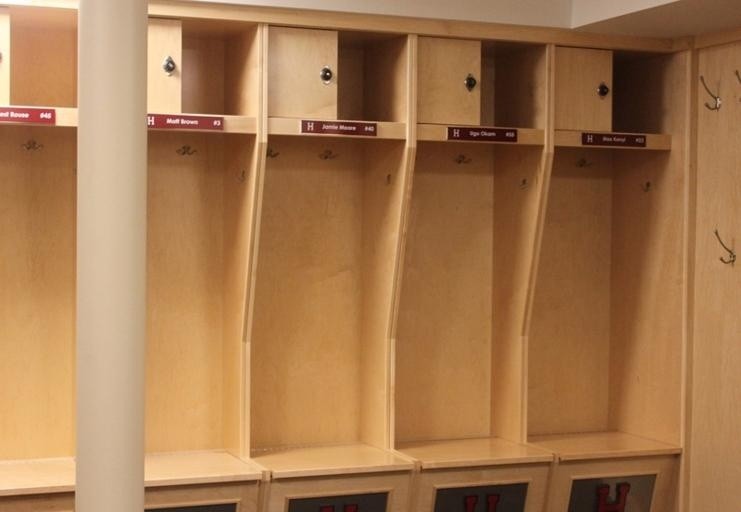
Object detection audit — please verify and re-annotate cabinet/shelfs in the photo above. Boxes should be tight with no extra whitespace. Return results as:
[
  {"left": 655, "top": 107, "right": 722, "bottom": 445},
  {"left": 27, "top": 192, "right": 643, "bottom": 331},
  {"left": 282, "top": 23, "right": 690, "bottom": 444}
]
[{"left": 0, "top": 0, "right": 688, "bottom": 512}]
